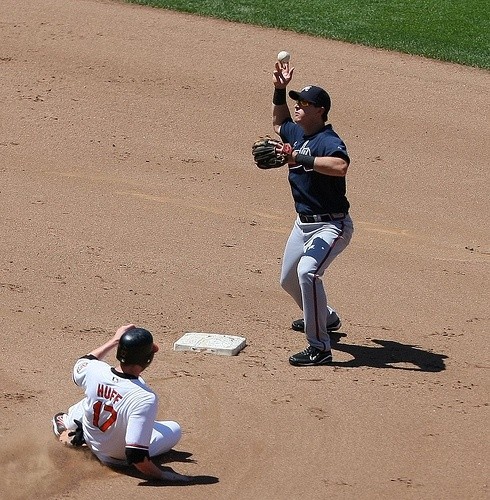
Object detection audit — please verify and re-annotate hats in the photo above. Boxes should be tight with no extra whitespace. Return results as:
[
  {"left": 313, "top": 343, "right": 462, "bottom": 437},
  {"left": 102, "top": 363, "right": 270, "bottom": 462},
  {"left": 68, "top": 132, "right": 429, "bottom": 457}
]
[{"left": 289, "top": 85, "right": 331, "bottom": 107}]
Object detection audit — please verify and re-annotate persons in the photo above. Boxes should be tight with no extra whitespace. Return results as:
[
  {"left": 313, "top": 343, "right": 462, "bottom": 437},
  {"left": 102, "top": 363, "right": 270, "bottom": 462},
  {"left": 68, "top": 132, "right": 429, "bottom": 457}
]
[
  {"left": 52, "top": 324, "right": 194, "bottom": 481},
  {"left": 251, "top": 60, "right": 354, "bottom": 368}
]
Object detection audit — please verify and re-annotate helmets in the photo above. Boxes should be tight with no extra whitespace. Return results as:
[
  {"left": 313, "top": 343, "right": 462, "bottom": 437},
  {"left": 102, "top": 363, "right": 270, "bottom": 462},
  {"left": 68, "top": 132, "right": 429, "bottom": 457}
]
[{"left": 116, "top": 328, "right": 158, "bottom": 367}]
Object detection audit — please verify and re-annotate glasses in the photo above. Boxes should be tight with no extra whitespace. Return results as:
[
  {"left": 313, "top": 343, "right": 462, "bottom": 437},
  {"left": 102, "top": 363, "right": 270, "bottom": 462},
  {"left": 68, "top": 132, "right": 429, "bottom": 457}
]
[{"left": 295, "top": 99, "right": 313, "bottom": 106}]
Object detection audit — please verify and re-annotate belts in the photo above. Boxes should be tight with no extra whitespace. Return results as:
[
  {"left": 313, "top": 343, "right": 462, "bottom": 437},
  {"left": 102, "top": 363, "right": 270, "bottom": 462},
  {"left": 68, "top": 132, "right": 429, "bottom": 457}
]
[{"left": 299, "top": 211, "right": 348, "bottom": 223}]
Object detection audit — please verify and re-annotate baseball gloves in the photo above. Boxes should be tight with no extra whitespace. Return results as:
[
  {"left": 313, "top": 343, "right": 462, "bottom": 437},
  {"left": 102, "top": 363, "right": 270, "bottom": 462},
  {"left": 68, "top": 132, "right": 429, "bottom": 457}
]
[{"left": 252, "top": 135, "right": 293, "bottom": 170}]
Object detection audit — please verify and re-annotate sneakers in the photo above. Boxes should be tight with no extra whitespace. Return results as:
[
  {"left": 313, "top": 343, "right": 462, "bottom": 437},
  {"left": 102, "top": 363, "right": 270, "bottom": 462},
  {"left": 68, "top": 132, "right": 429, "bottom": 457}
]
[
  {"left": 289, "top": 345, "right": 332, "bottom": 367},
  {"left": 52, "top": 413, "right": 67, "bottom": 437},
  {"left": 291, "top": 311, "right": 342, "bottom": 332}
]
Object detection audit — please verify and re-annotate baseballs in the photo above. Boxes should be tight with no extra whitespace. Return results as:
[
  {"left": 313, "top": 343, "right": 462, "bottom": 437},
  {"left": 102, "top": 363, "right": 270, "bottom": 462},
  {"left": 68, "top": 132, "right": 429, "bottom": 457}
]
[{"left": 278, "top": 51, "right": 290, "bottom": 63}]
